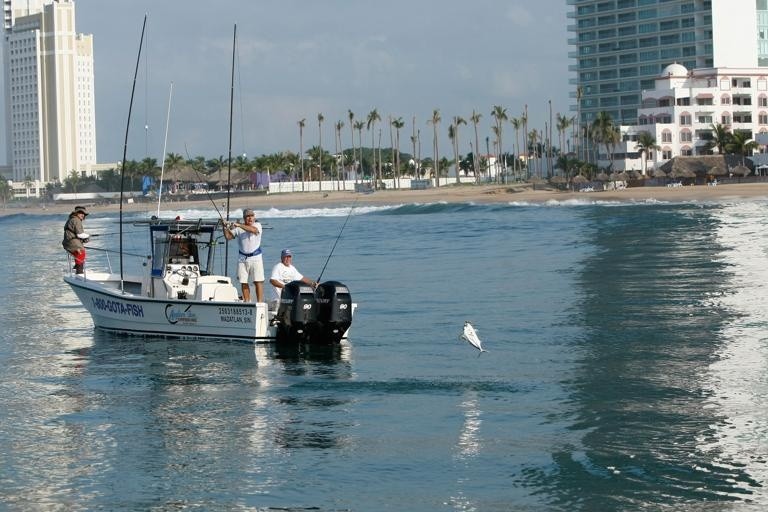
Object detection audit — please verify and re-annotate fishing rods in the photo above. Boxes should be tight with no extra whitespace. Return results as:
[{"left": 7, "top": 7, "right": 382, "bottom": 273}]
[
  {"left": 312, "top": 157, "right": 390, "bottom": 289},
  {"left": 91, "top": 228, "right": 152, "bottom": 236},
  {"left": 83, "top": 246, "right": 146, "bottom": 259},
  {"left": 182, "top": 140, "right": 236, "bottom": 240}
]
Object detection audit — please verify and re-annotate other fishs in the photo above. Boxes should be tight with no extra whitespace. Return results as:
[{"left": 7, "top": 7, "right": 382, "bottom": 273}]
[{"left": 459, "top": 320, "right": 489, "bottom": 357}]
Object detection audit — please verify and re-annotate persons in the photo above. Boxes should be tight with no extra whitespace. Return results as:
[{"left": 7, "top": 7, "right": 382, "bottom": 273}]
[
  {"left": 62, "top": 206, "right": 89, "bottom": 274},
  {"left": 270, "top": 249, "right": 320, "bottom": 299},
  {"left": 221, "top": 208, "right": 265, "bottom": 303}
]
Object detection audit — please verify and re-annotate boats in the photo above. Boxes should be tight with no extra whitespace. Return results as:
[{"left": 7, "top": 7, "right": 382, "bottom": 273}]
[{"left": 63, "top": 215, "right": 357, "bottom": 344}]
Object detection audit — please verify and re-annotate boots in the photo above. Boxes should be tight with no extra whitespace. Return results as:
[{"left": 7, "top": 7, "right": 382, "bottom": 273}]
[{"left": 74, "top": 264, "right": 83, "bottom": 274}]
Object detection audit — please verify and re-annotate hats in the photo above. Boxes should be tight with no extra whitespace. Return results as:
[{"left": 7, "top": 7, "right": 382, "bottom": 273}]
[
  {"left": 243, "top": 209, "right": 254, "bottom": 217},
  {"left": 72, "top": 206, "right": 89, "bottom": 215},
  {"left": 281, "top": 249, "right": 292, "bottom": 256}
]
